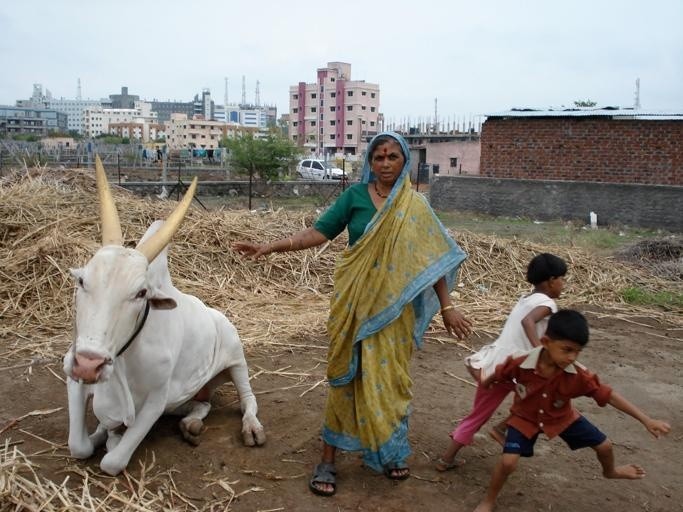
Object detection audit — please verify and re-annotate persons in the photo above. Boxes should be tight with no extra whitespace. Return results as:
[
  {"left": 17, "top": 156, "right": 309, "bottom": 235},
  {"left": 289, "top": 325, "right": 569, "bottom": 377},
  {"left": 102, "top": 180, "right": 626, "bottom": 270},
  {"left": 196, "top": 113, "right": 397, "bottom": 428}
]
[
  {"left": 232, "top": 131, "right": 476, "bottom": 497},
  {"left": 432, "top": 252, "right": 567, "bottom": 472},
  {"left": 156, "top": 146, "right": 162, "bottom": 160},
  {"left": 175, "top": 143, "right": 200, "bottom": 158},
  {"left": 472, "top": 308, "right": 671, "bottom": 512}
]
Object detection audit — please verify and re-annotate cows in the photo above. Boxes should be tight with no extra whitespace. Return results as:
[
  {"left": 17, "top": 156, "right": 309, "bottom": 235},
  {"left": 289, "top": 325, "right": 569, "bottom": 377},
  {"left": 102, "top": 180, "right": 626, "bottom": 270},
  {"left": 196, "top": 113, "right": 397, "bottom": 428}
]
[{"left": 62, "top": 154, "right": 266, "bottom": 477}]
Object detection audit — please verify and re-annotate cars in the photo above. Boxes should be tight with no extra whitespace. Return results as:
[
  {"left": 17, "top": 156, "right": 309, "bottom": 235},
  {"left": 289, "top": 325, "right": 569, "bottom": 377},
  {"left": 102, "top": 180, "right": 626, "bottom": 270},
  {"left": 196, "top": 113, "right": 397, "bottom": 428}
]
[{"left": 295, "top": 158, "right": 348, "bottom": 181}]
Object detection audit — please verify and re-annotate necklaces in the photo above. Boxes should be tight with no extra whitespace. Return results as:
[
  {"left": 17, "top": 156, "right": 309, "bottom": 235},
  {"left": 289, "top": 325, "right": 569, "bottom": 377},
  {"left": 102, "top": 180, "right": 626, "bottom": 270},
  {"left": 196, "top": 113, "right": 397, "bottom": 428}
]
[{"left": 374, "top": 181, "right": 391, "bottom": 204}]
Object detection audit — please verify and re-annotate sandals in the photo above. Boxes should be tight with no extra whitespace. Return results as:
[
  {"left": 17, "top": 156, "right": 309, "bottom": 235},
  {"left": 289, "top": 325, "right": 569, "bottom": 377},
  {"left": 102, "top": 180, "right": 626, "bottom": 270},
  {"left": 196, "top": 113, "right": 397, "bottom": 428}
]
[{"left": 437, "top": 459, "right": 464, "bottom": 471}]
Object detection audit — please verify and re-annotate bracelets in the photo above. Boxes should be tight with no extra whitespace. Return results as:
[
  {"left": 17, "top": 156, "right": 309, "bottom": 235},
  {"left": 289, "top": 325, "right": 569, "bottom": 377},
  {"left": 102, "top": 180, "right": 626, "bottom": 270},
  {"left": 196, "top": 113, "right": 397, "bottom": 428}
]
[
  {"left": 288, "top": 238, "right": 293, "bottom": 250},
  {"left": 440, "top": 305, "right": 454, "bottom": 311}
]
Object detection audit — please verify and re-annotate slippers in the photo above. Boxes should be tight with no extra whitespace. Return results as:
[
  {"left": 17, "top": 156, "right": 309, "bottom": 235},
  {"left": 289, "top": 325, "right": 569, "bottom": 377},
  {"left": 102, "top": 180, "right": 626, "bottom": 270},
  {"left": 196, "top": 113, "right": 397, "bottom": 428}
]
[
  {"left": 309, "top": 462, "right": 336, "bottom": 496},
  {"left": 384, "top": 459, "right": 409, "bottom": 480}
]
